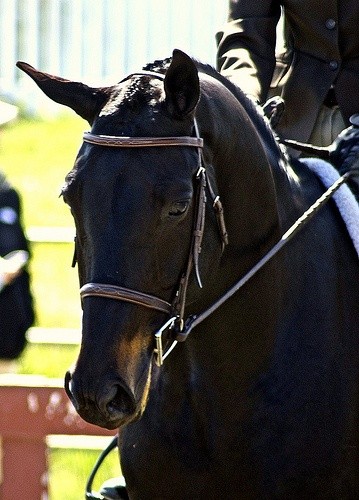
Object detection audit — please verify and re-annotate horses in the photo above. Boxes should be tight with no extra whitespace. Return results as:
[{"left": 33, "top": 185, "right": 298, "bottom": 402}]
[{"left": 16, "top": 48, "right": 359, "bottom": 500}]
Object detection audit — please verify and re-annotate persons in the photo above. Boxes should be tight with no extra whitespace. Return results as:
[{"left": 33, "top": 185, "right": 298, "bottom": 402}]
[
  {"left": 0, "top": 170, "right": 37, "bottom": 374},
  {"left": 216, "top": 0, "right": 359, "bottom": 199}
]
